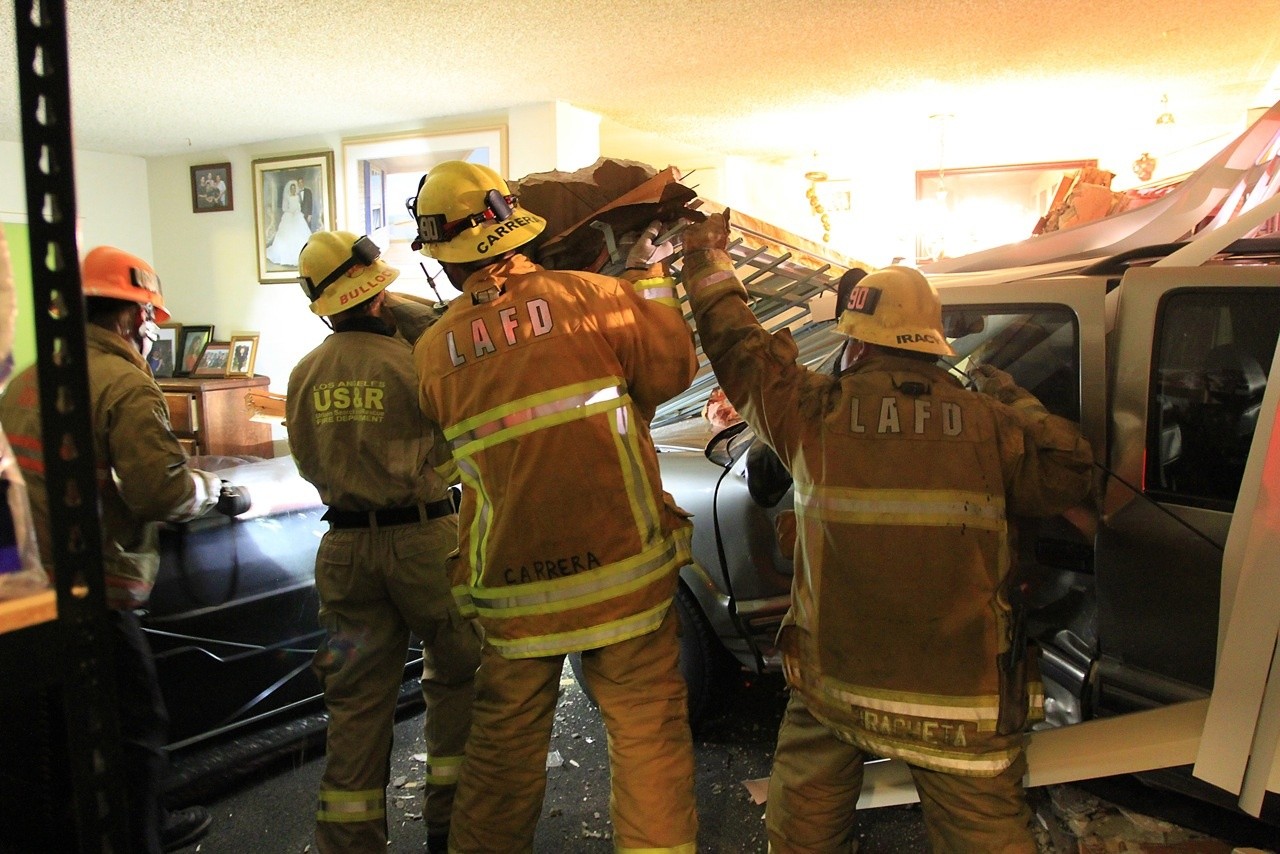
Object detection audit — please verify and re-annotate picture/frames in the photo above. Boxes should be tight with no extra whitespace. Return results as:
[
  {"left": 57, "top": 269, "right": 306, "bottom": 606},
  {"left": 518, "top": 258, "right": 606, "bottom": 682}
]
[
  {"left": 339, "top": 117, "right": 509, "bottom": 256},
  {"left": 187, "top": 161, "right": 234, "bottom": 214},
  {"left": 225, "top": 334, "right": 263, "bottom": 379},
  {"left": 189, "top": 340, "right": 230, "bottom": 379},
  {"left": 172, "top": 324, "right": 217, "bottom": 378},
  {"left": 147, "top": 321, "right": 184, "bottom": 377},
  {"left": 251, "top": 149, "right": 343, "bottom": 286}
]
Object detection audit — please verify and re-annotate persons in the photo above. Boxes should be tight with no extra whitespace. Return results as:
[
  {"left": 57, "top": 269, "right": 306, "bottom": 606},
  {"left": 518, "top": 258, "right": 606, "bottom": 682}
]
[
  {"left": 285, "top": 227, "right": 486, "bottom": 854},
  {"left": 681, "top": 209, "right": 1097, "bottom": 854},
  {"left": 200, "top": 347, "right": 248, "bottom": 372},
  {"left": 414, "top": 161, "right": 698, "bottom": 854},
  {"left": 196, "top": 171, "right": 226, "bottom": 208},
  {"left": 266, "top": 177, "right": 312, "bottom": 267},
  {"left": 149, "top": 347, "right": 165, "bottom": 372},
  {"left": 0, "top": 244, "right": 223, "bottom": 854}
]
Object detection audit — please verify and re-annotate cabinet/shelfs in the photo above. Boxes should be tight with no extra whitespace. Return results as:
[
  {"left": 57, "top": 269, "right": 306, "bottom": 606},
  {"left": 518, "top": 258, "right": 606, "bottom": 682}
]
[{"left": 157, "top": 372, "right": 273, "bottom": 459}]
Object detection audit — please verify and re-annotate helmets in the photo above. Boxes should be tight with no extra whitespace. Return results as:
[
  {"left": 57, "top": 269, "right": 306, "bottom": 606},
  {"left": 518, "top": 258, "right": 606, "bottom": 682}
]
[
  {"left": 51, "top": 247, "right": 171, "bottom": 321},
  {"left": 413, "top": 160, "right": 546, "bottom": 263},
  {"left": 831, "top": 265, "right": 957, "bottom": 356},
  {"left": 300, "top": 229, "right": 400, "bottom": 315}
]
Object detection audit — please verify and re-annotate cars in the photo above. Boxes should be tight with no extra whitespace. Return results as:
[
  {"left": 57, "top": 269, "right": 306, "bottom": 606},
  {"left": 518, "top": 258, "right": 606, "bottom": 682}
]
[{"left": 0, "top": 448, "right": 424, "bottom": 764}]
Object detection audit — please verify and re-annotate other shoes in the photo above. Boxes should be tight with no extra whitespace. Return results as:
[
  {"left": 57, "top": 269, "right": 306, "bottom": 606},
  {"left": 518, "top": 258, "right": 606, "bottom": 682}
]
[{"left": 111, "top": 806, "right": 212, "bottom": 854}]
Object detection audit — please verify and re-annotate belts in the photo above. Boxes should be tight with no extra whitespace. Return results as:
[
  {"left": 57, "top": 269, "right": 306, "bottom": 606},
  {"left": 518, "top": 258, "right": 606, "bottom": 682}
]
[{"left": 333, "top": 499, "right": 454, "bottom": 529}]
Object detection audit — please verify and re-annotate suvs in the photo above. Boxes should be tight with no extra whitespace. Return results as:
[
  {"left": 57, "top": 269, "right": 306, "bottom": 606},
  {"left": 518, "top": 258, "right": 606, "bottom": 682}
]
[{"left": 563, "top": 236, "right": 1280, "bottom": 829}]
